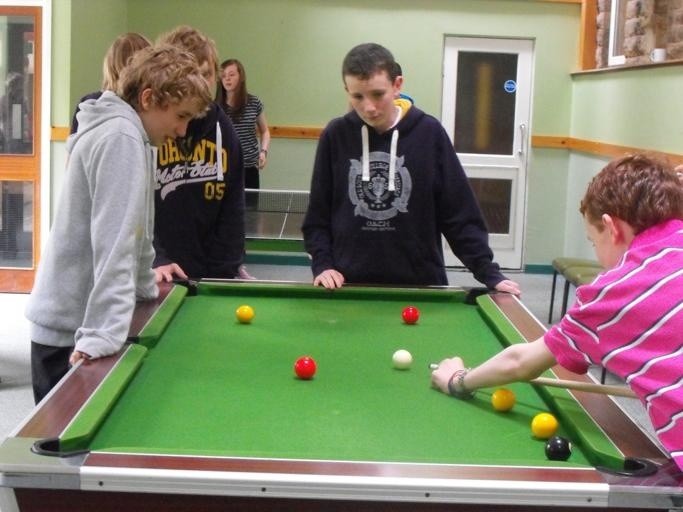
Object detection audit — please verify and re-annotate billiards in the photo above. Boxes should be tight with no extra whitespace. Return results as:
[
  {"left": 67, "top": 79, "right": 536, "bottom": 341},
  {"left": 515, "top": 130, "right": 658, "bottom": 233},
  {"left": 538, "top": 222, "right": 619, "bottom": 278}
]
[
  {"left": 490, "top": 389, "right": 515, "bottom": 412},
  {"left": 545, "top": 437, "right": 571, "bottom": 460},
  {"left": 530, "top": 412, "right": 560, "bottom": 440},
  {"left": 235, "top": 305, "right": 254, "bottom": 323},
  {"left": 393, "top": 350, "right": 413, "bottom": 370},
  {"left": 295, "top": 356, "right": 317, "bottom": 379},
  {"left": 403, "top": 306, "right": 419, "bottom": 324}
]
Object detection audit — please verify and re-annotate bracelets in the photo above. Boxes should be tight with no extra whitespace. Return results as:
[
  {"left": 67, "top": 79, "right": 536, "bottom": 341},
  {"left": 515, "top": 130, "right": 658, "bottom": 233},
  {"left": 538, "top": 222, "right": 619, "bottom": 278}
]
[
  {"left": 261, "top": 149, "right": 268, "bottom": 154},
  {"left": 448, "top": 367, "right": 477, "bottom": 400}
]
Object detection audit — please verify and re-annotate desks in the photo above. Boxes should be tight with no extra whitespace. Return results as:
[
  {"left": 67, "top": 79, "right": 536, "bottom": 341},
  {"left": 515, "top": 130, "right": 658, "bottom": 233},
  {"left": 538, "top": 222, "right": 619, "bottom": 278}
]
[{"left": 0, "top": 275, "right": 683, "bottom": 512}]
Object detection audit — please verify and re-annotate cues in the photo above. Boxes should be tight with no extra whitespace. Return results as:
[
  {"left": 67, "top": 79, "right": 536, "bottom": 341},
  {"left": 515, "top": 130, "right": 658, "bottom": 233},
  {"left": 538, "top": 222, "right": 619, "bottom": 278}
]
[{"left": 428, "top": 363, "right": 638, "bottom": 399}]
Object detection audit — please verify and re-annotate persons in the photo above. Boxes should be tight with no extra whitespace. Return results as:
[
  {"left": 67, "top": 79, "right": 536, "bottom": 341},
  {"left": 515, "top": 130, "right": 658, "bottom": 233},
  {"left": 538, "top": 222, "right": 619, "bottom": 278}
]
[
  {"left": 0, "top": 72, "right": 29, "bottom": 232},
  {"left": 300, "top": 43, "right": 522, "bottom": 301},
  {"left": 25, "top": 44, "right": 212, "bottom": 407},
  {"left": 431, "top": 154, "right": 683, "bottom": 470},
  {"left": 213, "top": 59, "right": 270, "bottom": 234},
  {"left": 151, "top": 26, "right": 256, "bottom": 282},
  {"left": 349, "top": 63, "right": 414, "bottom": 112},
  {"left": 70, "top": 32, "right": 151, "bottom": 134}
]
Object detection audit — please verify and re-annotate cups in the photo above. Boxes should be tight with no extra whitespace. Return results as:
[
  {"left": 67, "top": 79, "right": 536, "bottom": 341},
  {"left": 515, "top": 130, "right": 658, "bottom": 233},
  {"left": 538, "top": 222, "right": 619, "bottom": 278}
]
[{"left": 651, "top": 49, "right": 666, "bottom": 62}]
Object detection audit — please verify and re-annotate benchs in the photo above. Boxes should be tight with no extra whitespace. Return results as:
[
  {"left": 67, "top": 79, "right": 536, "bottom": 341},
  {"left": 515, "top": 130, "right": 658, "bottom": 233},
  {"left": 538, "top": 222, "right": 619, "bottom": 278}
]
[{"left": 547, "top": 255, "right": 619, "bottom": 384}]
